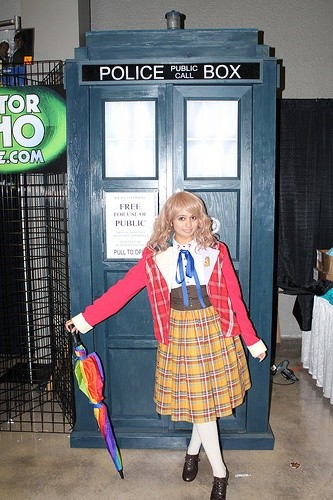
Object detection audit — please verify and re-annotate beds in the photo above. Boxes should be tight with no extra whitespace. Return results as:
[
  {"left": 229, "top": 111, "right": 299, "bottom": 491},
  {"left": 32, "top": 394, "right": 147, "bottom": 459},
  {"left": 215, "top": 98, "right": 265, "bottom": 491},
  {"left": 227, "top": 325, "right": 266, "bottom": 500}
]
[{"left": 301, "top": 295, "right": 333, "bottom": 405}]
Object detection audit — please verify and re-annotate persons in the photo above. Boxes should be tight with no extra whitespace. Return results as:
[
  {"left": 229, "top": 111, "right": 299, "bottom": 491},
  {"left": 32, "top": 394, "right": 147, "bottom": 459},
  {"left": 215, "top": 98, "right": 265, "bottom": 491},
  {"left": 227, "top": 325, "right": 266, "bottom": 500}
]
[
  {"left": 0, "top": 31, "right": 24, "bottom": 64},
  {"left": 64, "top": 191, "right": 268, "bottom": 500}
]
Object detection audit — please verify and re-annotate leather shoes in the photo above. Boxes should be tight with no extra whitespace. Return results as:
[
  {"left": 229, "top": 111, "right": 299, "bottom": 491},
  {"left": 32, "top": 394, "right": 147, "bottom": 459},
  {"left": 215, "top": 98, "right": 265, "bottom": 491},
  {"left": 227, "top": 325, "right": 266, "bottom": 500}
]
[
  {"left": 210, "top": 468, "right": 229, "bottom": 500},
  {"left": 182, "top": 448, "right": 201, "bottom": 482}
]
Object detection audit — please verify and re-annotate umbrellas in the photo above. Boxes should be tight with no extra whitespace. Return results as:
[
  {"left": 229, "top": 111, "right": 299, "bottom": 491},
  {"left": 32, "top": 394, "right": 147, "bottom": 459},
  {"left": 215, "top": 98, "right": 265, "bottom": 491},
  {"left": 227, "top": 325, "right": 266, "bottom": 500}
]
[{"left": 68, "top": 324, "right": 125, "bottom": 479}]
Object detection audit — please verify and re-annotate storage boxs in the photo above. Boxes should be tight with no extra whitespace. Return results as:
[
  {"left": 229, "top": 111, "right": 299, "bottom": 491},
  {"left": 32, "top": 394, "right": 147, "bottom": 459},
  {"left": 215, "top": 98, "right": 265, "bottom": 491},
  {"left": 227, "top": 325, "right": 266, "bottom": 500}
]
[{"left": 313, "top": 250, "right": 333, "bottom": 282}]
